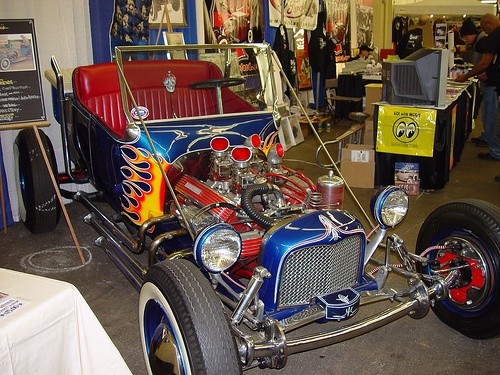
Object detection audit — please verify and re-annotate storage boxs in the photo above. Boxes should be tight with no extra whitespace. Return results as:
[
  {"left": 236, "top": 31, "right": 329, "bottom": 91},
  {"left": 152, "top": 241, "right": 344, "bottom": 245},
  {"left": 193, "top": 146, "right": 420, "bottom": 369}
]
[{"left": 340, "top": 83, "right": 382, "bottom": 189}]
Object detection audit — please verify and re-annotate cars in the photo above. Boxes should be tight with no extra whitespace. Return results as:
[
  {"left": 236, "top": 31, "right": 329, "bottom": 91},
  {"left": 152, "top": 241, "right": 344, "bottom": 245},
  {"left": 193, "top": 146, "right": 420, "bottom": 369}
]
[{"left": 11, "top": 42, "right": 500, "bottom": 372}]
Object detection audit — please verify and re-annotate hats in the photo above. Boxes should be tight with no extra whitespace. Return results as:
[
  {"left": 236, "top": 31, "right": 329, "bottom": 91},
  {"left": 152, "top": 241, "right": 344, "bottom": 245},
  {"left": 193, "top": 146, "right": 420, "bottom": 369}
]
[
  {"left": 360, "top": 45, "right": 373, "bottom": 51},
  {"left": 460, "top": 17, "right": 479, "bottom": 36}
]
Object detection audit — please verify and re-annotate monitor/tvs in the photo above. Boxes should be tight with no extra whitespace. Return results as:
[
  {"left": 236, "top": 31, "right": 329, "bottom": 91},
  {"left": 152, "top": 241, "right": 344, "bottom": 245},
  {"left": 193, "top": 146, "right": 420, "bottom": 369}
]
[{"left": 382, "top": 47, "right": 450, "bottom": 110}]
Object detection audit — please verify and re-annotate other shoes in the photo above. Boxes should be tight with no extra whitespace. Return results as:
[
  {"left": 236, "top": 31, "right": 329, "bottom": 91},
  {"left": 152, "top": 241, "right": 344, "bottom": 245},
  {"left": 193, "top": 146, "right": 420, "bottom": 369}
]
[
  {"left": 471, "top": 137, "right": 481, "bottom": 142},
  {"left": 495, "top": 175, "right": 500, "bottom": 182},
  {"left": 477, "top": 152, "right": 495, "bottom": 160},
  {"left": 475, "top": 139, "right": 488, "bottom": 146}
]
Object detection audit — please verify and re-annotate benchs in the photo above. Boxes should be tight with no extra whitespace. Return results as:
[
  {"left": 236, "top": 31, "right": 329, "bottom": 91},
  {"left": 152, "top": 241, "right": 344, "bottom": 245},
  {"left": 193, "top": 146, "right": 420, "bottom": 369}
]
[{"left": 72, "top": 60, "right": 258, "bottom": 140}]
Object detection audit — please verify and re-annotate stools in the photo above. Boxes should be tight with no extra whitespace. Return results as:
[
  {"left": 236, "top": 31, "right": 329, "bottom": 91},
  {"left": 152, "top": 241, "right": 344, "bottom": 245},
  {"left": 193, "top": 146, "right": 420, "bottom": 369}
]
[{"left": 325, "top": 96, "right": 361, "bottom": 129}]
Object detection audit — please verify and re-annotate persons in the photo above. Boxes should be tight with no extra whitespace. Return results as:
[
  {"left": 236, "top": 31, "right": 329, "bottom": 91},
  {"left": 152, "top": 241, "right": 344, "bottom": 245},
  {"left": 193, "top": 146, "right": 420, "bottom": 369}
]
[
  {"left": 351, "top": 44, "right": 373, "bottom": 60},
  {"left": 454, "top": 13, "right": 500, "bottom": 182}
]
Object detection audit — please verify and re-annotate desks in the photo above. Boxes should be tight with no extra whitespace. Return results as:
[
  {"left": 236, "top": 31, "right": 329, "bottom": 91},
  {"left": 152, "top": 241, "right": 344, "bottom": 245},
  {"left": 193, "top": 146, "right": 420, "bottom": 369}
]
[
  {"left": 373, "top": 76, "right": 483, "bottom": 190},
  {"left": 0, "top": 267, "right": 134, "bottom": 375}
]
